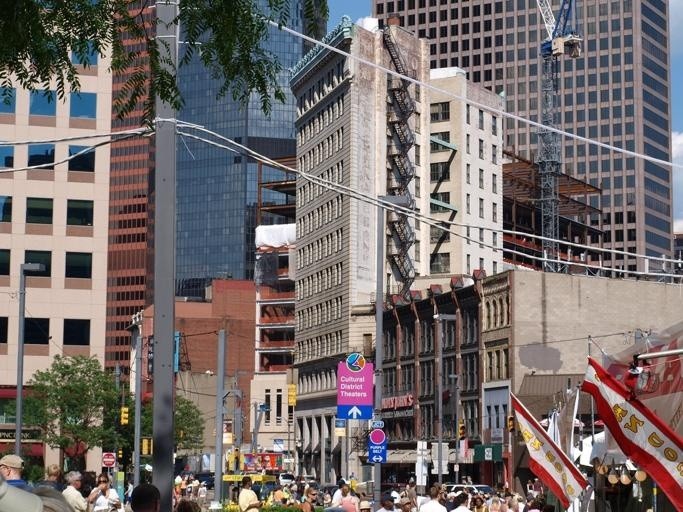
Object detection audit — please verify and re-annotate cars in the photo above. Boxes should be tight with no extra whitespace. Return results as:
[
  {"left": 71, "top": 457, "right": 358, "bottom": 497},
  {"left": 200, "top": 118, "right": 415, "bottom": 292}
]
[{"left": 195, "top": 471, "right": 494, "bottom": 503}]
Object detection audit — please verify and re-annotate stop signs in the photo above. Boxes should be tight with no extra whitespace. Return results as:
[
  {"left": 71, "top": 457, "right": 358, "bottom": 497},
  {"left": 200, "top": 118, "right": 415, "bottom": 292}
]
[{"left": 102, "top": 452, "right": 116, "bottom": 468}]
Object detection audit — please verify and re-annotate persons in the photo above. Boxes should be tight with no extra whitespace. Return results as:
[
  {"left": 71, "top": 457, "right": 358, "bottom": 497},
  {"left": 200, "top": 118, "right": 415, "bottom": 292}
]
[
  {"left": 0, "top": 454, "right": 160, "bottom": 512},
  {"left": 174, "top": 474, "right": 555, "bottom": 511}
]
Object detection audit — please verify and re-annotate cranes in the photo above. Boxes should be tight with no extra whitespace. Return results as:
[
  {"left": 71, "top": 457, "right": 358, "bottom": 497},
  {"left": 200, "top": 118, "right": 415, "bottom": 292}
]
[{"left": 535, "top": 0, "right": 583, "bottom": 274}]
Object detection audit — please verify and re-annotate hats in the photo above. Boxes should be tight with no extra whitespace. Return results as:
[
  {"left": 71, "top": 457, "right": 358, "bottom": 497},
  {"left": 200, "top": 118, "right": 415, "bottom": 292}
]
[
  {"left": 0, "top": 455, "right": 25, "bottom": 466},
  {"left": 400, "top": 498, "right": 410, "bottom": 507},
  {"left": 360, "top": 501, "right": 371, "bottom": 510}
]
